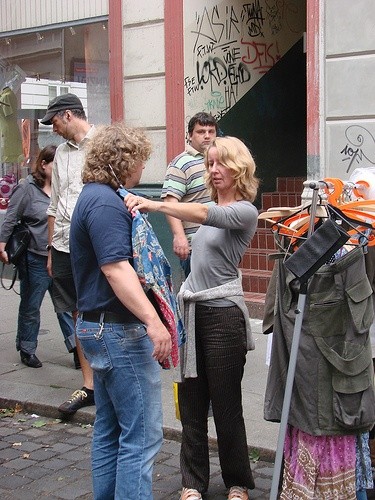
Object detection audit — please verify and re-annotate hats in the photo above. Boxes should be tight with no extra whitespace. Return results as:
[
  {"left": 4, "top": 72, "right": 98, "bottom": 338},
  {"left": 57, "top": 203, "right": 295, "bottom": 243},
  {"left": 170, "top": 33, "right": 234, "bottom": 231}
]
[{"left": 40, "top": 93, "right": 83, "bottom": 125}]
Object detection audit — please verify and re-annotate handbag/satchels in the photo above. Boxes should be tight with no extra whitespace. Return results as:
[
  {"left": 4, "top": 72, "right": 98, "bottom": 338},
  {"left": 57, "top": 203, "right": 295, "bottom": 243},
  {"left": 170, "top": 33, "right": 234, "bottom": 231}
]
[
  {"left": 1, "top": 223, "right": 31, "bottom": 295},
  {"left": 283, "top": 182, "right": 351, "bottom": 283}
]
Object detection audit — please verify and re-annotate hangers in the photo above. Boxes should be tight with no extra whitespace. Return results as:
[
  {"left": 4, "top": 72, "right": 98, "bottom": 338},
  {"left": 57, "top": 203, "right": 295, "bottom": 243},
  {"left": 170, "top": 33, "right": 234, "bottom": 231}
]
[{"left": 258, "top": 178, "right": 375, "bottom": 252}]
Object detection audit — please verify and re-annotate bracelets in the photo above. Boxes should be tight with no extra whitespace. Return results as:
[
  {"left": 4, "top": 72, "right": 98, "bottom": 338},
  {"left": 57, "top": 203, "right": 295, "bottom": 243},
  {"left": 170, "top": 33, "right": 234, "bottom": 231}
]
[{"left": 46, "top": 245, "right": 52, "bottom": 252}]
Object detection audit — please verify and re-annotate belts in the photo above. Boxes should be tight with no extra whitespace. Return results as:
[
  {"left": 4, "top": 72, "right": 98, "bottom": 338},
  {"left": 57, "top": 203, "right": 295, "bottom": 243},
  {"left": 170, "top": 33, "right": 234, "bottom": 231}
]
[{"left": 79, "top": 311, "right": 139, "bottom": 324}]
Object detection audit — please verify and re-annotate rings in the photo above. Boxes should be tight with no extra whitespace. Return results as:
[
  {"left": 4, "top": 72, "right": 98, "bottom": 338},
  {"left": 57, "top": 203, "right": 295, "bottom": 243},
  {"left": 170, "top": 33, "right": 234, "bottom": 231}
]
[{"left": 137, "top": 199, "right": 142, "bottom": 204}]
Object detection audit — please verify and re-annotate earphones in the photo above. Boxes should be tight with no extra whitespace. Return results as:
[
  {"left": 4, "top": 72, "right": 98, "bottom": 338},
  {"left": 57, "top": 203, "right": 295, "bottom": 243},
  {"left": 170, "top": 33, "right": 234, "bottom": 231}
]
[{"left": 68, "top": 114, "right": 70, "bottom": 118}]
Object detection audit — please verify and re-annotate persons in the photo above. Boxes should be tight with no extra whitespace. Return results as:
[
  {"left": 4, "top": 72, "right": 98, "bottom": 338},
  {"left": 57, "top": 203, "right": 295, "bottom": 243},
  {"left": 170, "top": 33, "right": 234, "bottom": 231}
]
[
  {"left": 160, "top": 112, "right": 218, "bottom": 278},
  {"left": 70, "top": 123, "right": 172, "bottom": 500},
  {"left": 124, "top": 136, "right": 260, "bottom": 500},
  {"left": 0, "top": 144, "right": 82, "bottom": 368},
  {"left": 0, "top": 86, "right": 42, "bottom": 168},
  {"left": 40, "top": 92, "right": 99, "bottom": 414}
]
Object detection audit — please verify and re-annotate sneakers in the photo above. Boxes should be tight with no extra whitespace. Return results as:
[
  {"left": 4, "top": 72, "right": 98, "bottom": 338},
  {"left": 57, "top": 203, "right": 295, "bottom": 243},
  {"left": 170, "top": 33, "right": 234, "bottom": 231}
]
[
  {"left": 178, "top": 487, "right": 202, "bottom": 500},
  {"left": 227, "top": 486, "right": 249, "bottom": 500},
  {"left": 58, "top": 387, "right": 95, "bottom": 413}
]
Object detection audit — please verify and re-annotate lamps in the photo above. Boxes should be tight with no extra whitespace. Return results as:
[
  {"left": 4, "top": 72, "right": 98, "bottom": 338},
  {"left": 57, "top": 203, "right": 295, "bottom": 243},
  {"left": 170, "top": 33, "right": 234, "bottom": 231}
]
[
  {"left": 68, "top": 27, "right": 77, "bottom": 36},
  {"left": 35, "top": 32, "right": 44, "bottom": 41}
]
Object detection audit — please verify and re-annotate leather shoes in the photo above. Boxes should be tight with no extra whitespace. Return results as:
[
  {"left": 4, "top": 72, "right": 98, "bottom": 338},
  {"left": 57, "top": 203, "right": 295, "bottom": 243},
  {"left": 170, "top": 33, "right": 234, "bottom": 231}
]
[
  {"left": 20, "top": 350, "right": 42, "bottom": 368},
  {"left": 74, "top": 356, "right": 81, "bottom": 369}
]
